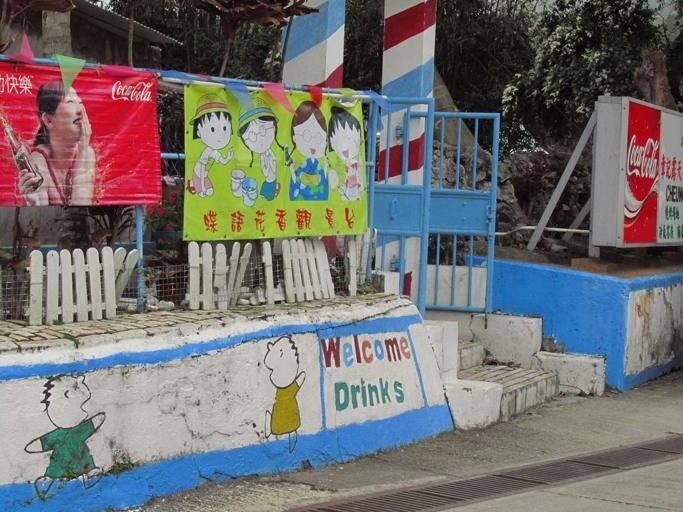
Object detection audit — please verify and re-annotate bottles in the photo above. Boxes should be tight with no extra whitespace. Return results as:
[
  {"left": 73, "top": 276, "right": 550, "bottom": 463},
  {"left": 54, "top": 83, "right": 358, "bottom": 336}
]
[{"left": 3, "top": 124, "right": 44, "bottom": 191}]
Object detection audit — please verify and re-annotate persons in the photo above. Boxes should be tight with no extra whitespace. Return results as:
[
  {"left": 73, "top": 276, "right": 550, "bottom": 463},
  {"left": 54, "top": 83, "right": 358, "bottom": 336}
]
[{"left": 18, "top": 81, "right": 96, "bottom": 205}]
